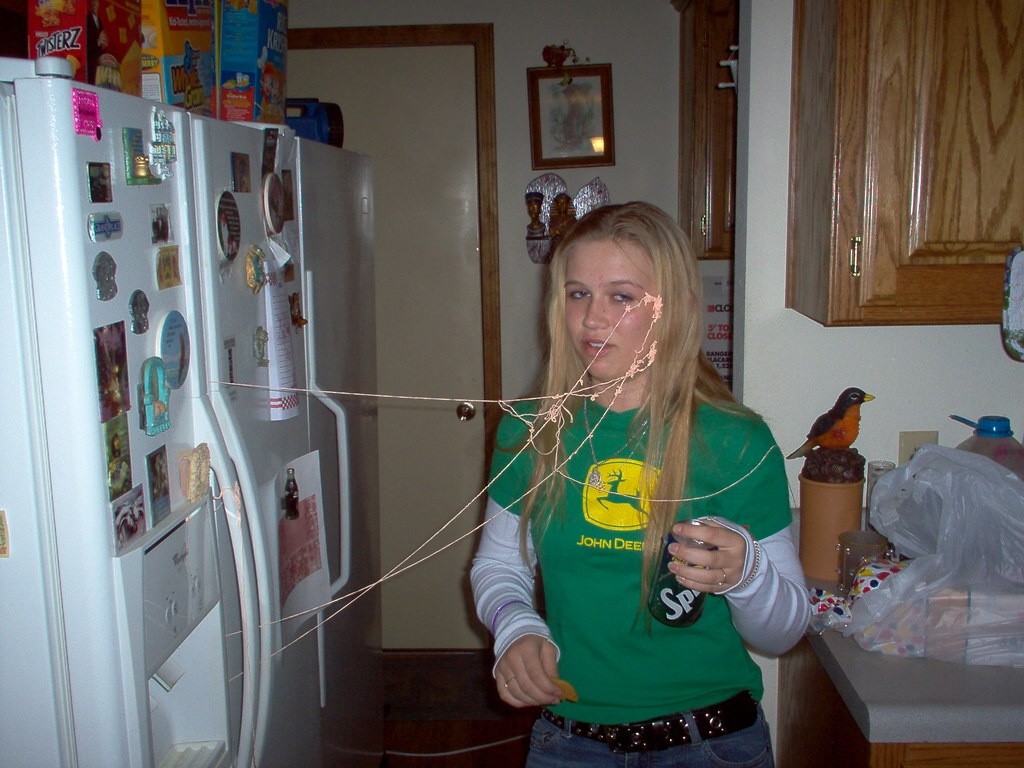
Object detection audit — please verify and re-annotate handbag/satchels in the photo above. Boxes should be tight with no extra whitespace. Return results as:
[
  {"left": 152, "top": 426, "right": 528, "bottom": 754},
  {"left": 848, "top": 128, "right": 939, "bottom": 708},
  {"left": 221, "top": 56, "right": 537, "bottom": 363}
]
[{"left": 823, "top": 442, "right": 1023, "bottom": 665}]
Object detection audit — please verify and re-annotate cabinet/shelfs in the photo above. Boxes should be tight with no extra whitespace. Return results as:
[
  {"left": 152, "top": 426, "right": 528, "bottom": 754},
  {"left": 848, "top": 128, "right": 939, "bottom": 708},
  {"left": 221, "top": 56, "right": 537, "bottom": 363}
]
[
  {"left": 669, "top": 0, "right": 740, "bottom": 261},
  {"left": 785, "top": 0, "right": 1024, "bottom": 329},
  {"left": 775, "top": 509, "right": 1024, "bottom": 768}
]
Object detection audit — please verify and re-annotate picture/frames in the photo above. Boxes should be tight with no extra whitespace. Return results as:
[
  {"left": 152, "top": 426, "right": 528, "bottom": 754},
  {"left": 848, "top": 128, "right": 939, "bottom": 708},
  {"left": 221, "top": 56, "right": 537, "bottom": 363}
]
[{"left": 526, "top": 63, "right": 616, "bottom": 170}]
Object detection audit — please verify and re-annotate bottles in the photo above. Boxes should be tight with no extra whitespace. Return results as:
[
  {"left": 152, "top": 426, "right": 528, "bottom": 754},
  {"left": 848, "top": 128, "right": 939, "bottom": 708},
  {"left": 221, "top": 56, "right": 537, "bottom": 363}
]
[{"left": 948, "top": 414, "right": 1024, "bottom": 482}]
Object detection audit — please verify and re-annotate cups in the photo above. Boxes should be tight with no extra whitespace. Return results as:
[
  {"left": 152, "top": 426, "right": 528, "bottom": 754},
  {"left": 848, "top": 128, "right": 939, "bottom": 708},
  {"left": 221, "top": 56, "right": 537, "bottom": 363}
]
[
  {"left": 837, "top": 530, "right": 887, "bottom": 597},
  {"left": 866, "top": 460, "right": 895, "bottom": 529}
]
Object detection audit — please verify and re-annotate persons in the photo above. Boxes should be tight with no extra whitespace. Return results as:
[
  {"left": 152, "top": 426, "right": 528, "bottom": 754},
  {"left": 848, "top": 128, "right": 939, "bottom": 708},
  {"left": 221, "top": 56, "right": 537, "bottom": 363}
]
[{"left": 470, "top": 201, "right": 811, "bottom": 768}]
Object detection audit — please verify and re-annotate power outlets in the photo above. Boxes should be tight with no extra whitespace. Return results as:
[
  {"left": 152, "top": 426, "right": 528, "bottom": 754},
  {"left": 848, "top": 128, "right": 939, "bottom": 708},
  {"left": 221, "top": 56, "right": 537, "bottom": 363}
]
[{"left": 898, "top": 431, "right": 938, "bottom": 467}]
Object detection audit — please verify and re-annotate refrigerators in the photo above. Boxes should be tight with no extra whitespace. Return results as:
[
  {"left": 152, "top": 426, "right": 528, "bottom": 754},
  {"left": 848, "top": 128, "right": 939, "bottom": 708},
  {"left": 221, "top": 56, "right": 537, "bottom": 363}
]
[{"left": 1, "top": 73, "right": 376, "bottom": 768}]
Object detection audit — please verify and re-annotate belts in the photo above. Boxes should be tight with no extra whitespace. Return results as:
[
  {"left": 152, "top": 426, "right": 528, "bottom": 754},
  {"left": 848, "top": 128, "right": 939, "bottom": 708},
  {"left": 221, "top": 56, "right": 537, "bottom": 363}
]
[{"left": 542, "top": 691, "right": 758, "bottom": 754}]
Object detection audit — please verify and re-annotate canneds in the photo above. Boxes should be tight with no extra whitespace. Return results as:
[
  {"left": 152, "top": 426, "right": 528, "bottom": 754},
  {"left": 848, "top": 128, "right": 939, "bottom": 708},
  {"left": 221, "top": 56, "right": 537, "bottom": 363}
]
[{"left": 647, "top": 518, "right": 718, "bottom": 627}]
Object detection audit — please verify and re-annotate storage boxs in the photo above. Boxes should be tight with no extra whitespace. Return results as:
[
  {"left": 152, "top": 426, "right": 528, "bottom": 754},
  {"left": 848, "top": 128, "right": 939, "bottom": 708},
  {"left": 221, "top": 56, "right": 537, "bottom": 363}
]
[
  {"left": 26, "top": 0, "right": 290, "bottom": 124},
  {"left": 927, "top": 585, "right": 1024, "bottom": 667}
]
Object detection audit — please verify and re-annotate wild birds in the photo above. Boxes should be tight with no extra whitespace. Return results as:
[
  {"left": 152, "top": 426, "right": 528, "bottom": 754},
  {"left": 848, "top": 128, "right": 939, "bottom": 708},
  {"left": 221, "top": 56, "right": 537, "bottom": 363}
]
[{"left": 784, "top": 386, "right": 877, "bottom": 461}]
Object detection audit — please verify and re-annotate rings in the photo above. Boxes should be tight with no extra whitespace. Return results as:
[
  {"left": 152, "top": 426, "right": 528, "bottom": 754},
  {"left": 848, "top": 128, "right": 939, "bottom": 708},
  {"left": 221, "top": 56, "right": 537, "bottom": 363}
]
[
  {"left": 717, "top": 568, "right": 726, "bottom": 586},
  {"left": 505, "top": 677, "right": 517, "bottom": 688}
]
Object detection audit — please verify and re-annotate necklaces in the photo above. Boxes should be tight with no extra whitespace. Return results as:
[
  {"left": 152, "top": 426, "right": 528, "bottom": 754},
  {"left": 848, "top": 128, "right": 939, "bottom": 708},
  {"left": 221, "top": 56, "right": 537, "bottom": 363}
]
[{"left": 584, "top": 396, "right": 650, "bottom": 493}]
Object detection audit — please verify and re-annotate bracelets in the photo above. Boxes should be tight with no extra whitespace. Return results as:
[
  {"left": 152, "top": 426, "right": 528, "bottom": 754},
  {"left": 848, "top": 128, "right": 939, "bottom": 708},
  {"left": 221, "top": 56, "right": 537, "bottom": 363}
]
[
  {"left": 490, "top": 600, "right": 533, "bottom": 636},
  {"left": 734, "top": 525, "right": 762, "bottom": 592}
]
